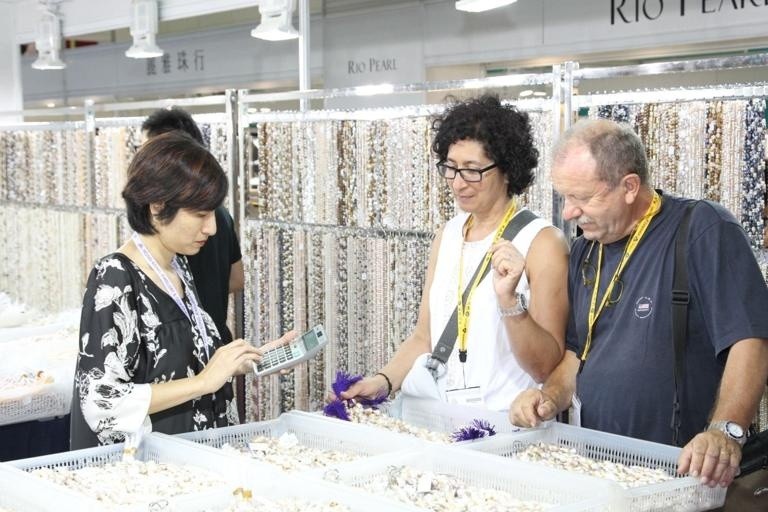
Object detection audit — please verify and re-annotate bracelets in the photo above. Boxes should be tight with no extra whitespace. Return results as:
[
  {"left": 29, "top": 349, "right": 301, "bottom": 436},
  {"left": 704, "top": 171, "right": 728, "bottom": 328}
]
[{"left": 375, "top": 370, "right": 393, "bottom": 400}]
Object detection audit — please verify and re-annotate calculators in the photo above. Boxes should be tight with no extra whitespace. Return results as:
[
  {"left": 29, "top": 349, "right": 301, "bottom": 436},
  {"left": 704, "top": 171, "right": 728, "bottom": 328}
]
[{"left": 252, "top": 323, "right": 330, "bottom": 377}]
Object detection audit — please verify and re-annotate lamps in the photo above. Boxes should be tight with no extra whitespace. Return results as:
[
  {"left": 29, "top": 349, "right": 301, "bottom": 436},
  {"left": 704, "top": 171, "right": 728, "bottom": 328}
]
[
  {"left": 124, "top": 1, "right": 166, "bottom": 60},
  {"left": 32, "top": 0, "right": 67, "bottom": 73},
  {"left": 250, "top": 0, "right": 303, "bottom": 42}
]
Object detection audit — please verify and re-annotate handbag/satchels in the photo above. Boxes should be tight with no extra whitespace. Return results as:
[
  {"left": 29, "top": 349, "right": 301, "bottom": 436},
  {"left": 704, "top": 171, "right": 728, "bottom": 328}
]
[{"left": 674, "top": 421, "right": 768, "bottom": 511}]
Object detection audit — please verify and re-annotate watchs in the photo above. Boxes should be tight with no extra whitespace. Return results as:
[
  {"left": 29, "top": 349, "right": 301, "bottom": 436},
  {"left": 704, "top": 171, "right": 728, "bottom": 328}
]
[
  {"left": 702, "top": 416, "right": 748, "bottom": 448},
  {"left": 495, "top": 290, "right": 532, "bottom": 321}
]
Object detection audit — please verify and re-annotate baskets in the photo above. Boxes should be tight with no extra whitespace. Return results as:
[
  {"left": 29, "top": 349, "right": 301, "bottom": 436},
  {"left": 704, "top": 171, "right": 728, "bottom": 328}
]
[
  {"left": 0, "top": 403, "right": 727, "bottom": 512},
  {"left": 2, "top": 277, "right": 75, "bottom": 426},
  {"left": 7, "top": 397, "right": 727, "bottom": 510}
]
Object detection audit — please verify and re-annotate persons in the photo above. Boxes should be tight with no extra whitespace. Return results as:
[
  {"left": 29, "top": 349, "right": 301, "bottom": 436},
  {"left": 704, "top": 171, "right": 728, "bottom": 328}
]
[
  {"left": 508, "top": 115, "right": 768, "bottom": 489},
  {"left": 66, "top": 131, "right": 298, "bottom": 450},
  {"left": 326, "top": 89, "right": 572, "bottom": 409},
  {"left": 138, "top": 104, "right": 247, "bottom": 349}
]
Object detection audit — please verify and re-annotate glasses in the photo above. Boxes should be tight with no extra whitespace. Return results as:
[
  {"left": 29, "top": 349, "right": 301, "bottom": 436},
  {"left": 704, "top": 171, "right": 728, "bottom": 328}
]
[{"left": 436, "top": 159, "right": 497, "bottom": 183}]
